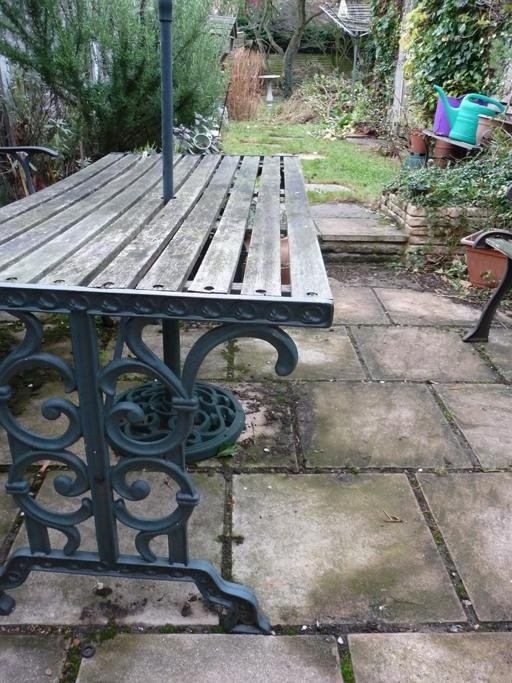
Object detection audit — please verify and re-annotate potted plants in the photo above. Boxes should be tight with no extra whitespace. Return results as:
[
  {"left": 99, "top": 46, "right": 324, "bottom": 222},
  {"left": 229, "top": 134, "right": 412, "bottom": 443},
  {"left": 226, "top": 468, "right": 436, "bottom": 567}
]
[{"left": 462, "top": 163, "right": 511, "bottom": 293}]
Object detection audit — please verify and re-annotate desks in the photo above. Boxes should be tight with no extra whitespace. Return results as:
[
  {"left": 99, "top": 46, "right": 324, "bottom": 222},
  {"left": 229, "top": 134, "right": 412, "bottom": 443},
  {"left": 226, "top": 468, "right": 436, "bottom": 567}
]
[{"left": 1, "top": 153, "right": 333, "bottom": 634}]
